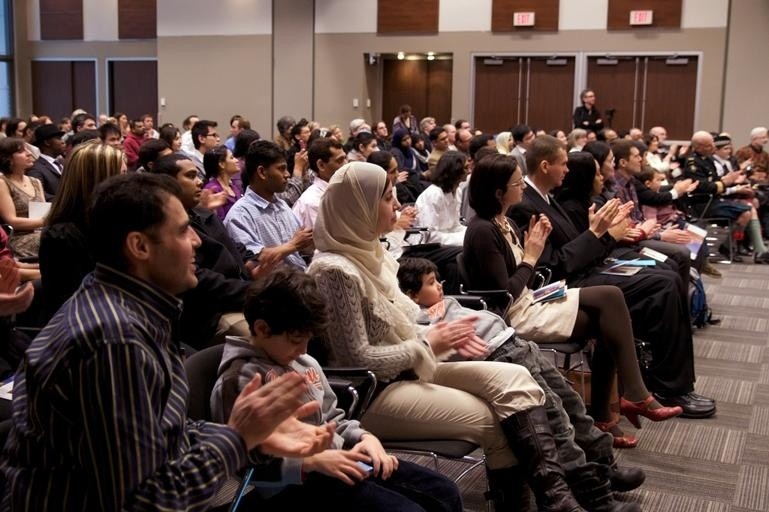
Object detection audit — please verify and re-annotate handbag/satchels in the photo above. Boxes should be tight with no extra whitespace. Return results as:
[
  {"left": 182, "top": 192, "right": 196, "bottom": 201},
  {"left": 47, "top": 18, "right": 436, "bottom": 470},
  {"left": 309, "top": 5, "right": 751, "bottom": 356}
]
[{"left": 687, "top": 267, "right": 706, "bottom": 333}]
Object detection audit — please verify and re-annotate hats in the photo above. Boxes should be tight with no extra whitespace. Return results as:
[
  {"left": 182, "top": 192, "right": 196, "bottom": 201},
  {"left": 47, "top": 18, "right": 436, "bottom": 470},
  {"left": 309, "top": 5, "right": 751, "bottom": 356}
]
[
  {"left": 714, "top": 135, "right": 730, "bottom": 147},
  {"left": 31, "top": 124, "right": 67, "bottom": 145},
  {"left": 278, "top": 115, "right": 296, "bottom": 134}
]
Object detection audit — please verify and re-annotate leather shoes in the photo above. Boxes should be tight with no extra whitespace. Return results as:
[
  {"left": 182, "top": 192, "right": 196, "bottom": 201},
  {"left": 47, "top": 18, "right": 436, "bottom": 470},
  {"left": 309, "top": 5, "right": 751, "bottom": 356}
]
[
  {"left": 652, "top": 391, "right": 716, "bottom": 418},
  {"left": 719, "top": 238, "right": 769, "bottom": 264}
]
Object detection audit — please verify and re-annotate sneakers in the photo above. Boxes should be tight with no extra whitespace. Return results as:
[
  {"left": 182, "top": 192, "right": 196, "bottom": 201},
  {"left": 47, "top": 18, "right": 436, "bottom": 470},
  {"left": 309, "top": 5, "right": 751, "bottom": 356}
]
[{"left": 704, "top": 262, "right": 722, "bottom": 278}]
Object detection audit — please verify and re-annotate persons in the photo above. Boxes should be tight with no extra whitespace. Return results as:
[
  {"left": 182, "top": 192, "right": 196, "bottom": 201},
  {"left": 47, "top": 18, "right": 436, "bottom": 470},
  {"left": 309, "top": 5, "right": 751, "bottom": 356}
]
[
  {"left": 573, "top": 88, "right": 605, "bottom": 133},
  {"left": 1, "top": 106, "right": 767, "bottom": 512}
]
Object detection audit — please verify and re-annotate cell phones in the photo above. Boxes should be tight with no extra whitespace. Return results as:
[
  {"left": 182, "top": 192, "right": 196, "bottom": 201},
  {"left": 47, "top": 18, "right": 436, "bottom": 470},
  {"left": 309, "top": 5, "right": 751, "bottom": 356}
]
[{"left": 353, "top": 458, "right": 374, "bottom": 475}]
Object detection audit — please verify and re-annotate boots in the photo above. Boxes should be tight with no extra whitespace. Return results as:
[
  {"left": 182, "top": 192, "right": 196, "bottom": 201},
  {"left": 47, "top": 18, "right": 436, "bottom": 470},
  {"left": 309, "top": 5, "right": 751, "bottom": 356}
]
[
  {"left": 597, "top": 456, "right": 646, "bottom": 490},
  {"left": 575, "top": 480, "right": 642, "bottom": 512},
  {"left": 501, "top": 405, "right": 589, "bottom": 512},
  {"left": 483, "top": 457, "right": 529, "bottom": 512}
]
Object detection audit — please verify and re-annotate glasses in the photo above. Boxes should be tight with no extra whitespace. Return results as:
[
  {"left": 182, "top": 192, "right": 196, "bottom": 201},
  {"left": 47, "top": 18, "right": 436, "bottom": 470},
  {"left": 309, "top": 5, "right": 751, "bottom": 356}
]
[
  {"left": 201, "top": 132, "right": 218, "bottom": 138},
  {"left": 506, "top": 171, "right": 527, "bottom": 188},
  {"left": 603, "top": 156, "right": 615, "bottom": 167}
]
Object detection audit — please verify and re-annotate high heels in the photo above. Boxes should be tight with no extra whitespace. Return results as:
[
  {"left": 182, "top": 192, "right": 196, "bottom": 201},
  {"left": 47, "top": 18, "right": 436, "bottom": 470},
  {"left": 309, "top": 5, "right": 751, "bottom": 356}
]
[
  {"left": 594, "top": 420, "right": 638, "bottom": 447},
  {"left": 619, "top": 395, "right": 683, "bottom": 429}
]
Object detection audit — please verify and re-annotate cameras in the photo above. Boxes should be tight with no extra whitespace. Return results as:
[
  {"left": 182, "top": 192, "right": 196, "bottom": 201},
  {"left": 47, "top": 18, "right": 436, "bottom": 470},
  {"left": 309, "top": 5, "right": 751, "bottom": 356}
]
[{"left": 605, "top": 108, "right": 616, "bottom": 116}]
